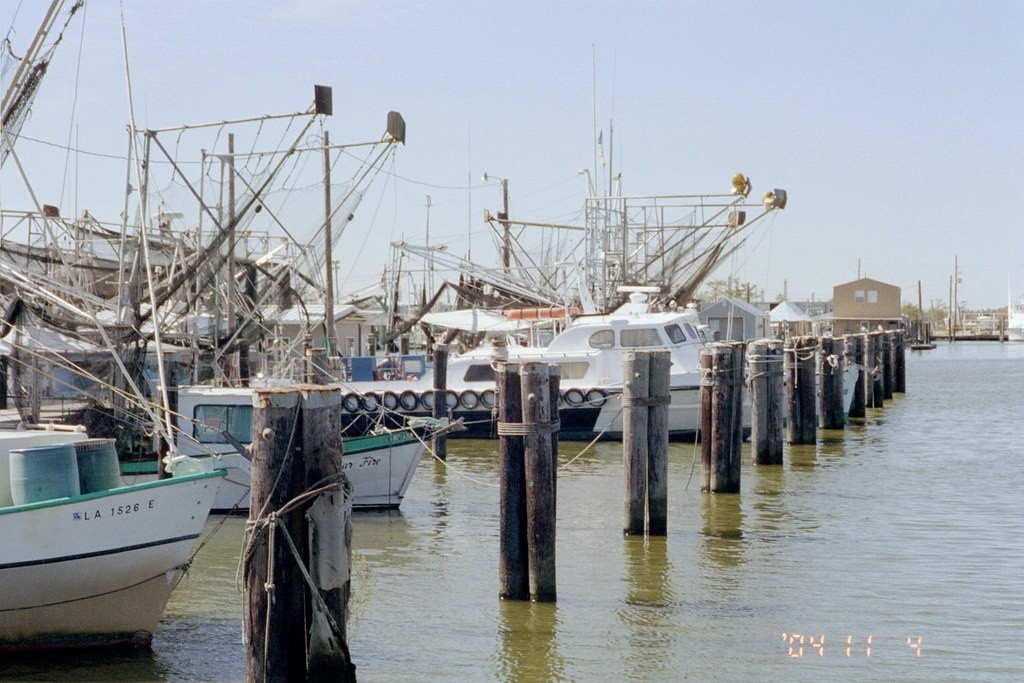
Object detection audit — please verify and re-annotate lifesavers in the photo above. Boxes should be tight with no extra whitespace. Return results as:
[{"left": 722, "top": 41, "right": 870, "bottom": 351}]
[{"left": 377, "top": 360, "right": 402, "bottom": 380}]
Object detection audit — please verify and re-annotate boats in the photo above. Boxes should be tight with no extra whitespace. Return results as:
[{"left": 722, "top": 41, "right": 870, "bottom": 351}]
[{"left": 1007, "top": 313, "right": 1024, "bottom": 342}]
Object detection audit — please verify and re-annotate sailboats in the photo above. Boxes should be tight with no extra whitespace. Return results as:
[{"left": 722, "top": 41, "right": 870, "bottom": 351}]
[{"left": 0, "top": 0, "right": 858, "bottom": 658}]
